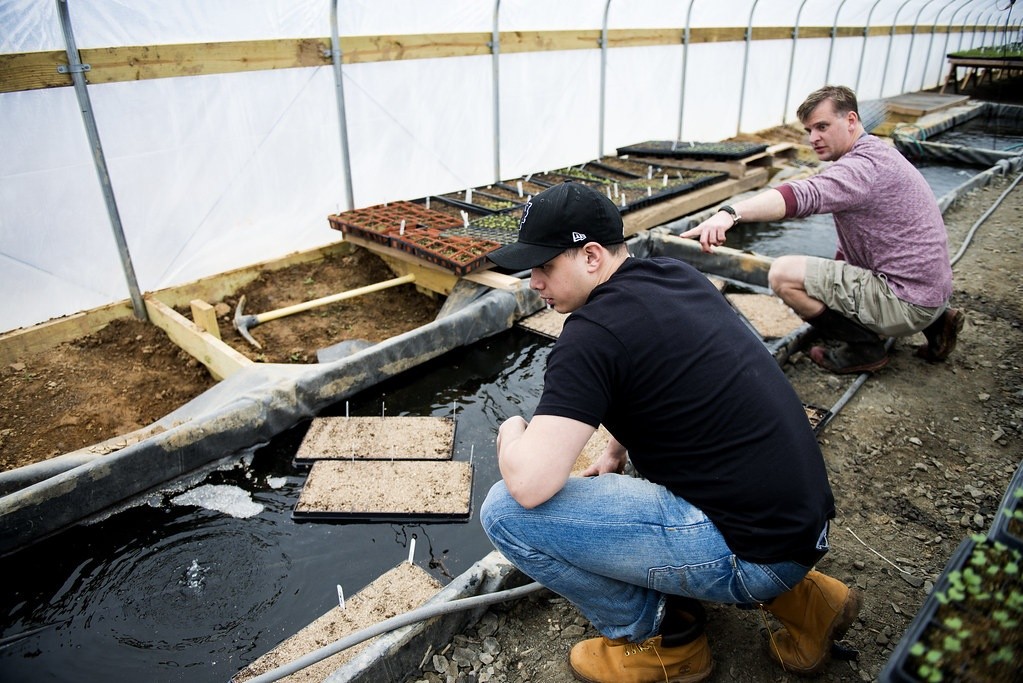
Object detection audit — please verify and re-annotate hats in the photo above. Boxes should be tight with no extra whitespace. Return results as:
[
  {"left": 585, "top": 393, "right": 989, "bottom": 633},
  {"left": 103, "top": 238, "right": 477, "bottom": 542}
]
[{"left": 486, "top": 180, "right": 624, "bottom": 271}]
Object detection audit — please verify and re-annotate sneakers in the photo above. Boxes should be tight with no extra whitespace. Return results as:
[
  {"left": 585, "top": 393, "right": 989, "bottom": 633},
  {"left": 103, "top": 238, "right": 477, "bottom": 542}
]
[
  {"left": 570, "top": 626, "right": 713, "bottom": 683},
  {"left": 768, "top": 570, "right": 863, "bottom": 673}
]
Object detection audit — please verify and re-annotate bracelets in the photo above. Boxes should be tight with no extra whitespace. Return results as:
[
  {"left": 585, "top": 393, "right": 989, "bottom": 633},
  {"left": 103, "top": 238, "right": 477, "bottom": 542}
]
[{"left": 718, "top": 205, "right": 740, "bottom": 226}]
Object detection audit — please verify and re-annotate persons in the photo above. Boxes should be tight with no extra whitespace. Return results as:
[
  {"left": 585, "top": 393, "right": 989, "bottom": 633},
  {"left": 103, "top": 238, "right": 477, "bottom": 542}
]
[
  {"left": 480, "top": 178, "right": 863, "bottom": 683},
  {"left": 678, "top": 85, "right": 965, "bottom": 378}
]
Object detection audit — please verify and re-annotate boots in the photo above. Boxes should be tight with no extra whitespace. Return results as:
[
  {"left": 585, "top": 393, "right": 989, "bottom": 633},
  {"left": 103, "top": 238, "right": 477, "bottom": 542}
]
[
  {"left": 918, "top": 307, "right": 965, "bottom": 365},
  {"left": 802, "top": 304, "right": 889, "bottom": 374}
]
